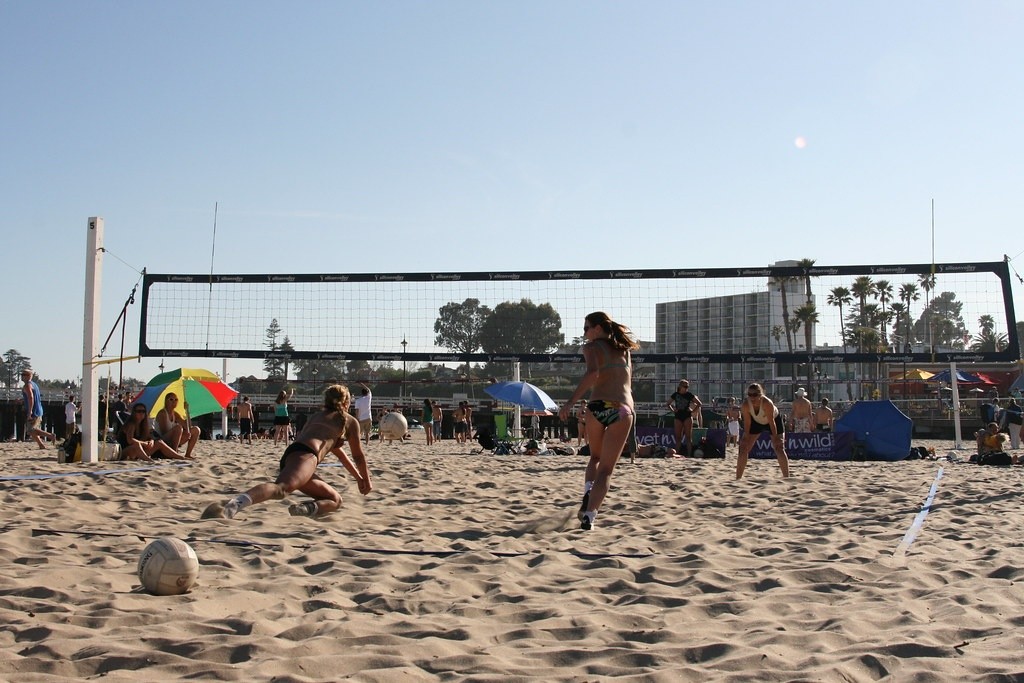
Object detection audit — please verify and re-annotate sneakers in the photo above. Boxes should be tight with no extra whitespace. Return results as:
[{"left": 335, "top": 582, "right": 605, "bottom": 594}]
[{"left": 578, "top": 481, "right": 598, "bottom": 530}]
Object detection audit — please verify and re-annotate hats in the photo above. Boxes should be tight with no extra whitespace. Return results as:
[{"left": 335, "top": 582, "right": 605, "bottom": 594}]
[{"left": 794, "top": 387, "right": 808, "bottom": 396}]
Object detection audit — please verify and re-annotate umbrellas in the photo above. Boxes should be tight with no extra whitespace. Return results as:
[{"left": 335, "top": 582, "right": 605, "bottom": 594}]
[
  {"left": 835, "top": 400, "right": 912, "bottom": 462},
  {"left": 482, "top": 378, "right": 560, "bottom": 434},
  {"left": 889, "top": 368, "right": 1002, "bottom": 399},
  {"left": 126, "top": 368, "right": 239, "bottom": 439}
]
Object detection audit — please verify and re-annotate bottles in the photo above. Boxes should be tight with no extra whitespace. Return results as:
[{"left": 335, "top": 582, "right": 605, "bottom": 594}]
[{"left": 58, "top": 446, "right": 65, "bottom": 464}]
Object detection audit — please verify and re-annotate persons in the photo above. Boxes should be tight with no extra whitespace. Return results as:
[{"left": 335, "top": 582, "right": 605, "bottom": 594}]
[
  {"left": 224, "top": 383, "right": 372, "bottom": 519},
  {"left": 666, "top": 379, "right": 702, "bottom": 457},
  {"left": 689, "top": 388, "right": 833, "bottom": 447},
  {"left": 736, "top": 382, "right": 789, "bottom": 480},
  {"left": 21, "top": 369, "right": 56, "bottom": 449},
  {"left": 63, "top": 383, "right": 589, "bottom": 461},
  {"left": 976, "top": 386, "right": 1024, "bottom": 464},
  {"left": 557, "top": 312, "right": 641, "bottom": 531}
]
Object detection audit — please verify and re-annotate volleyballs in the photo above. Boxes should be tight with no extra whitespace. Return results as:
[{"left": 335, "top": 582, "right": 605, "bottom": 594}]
[
  {"left": 379, "top": 413, "right": 407, "bottom": 440},
  {"left": 948, "top": 452, "right": 957, "bottom": 460},
  {"left": 139, "top": 537, "right": 198, "bottom": 595}
]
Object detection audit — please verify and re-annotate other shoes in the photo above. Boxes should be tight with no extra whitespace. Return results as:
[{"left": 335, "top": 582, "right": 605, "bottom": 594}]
[
  {"left": 289, "top": 502, "right": 315, "bottom": 516},
  {"left": 52, "top": 433, "right": 56, "bottom": 445}
]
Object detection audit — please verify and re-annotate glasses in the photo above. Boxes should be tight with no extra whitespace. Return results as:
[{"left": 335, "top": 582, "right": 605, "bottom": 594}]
[
  {"left": 748, "top": 393, "right": 759, "bottom": 397},
  {"left": 682, "top": 385, "right": 689, "bottom": 389},
  {"left": 584, "top": 324, "right": 595, "bottom": 332},
  {"left": 20, "top": 374, "right": 29, "bottom": 377},
  {"left": 169, "top": 398, "right": 178, "bottom": 401},
  {"left": 135, "top": 409, "right": 145, "bottom": 414}
]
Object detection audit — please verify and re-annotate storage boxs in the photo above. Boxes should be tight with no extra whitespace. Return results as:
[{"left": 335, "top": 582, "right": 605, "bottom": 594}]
[{"left": 99, "top": 440, "right": 121, "bottom": 460}]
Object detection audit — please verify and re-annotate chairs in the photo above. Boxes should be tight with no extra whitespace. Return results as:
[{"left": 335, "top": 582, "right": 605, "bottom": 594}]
[
  {"left": 494, "top": 413, "right": 527, "bottom": 455},
  {"left": 471, "top": 412, "right": 500, "bottom": 454}
]
